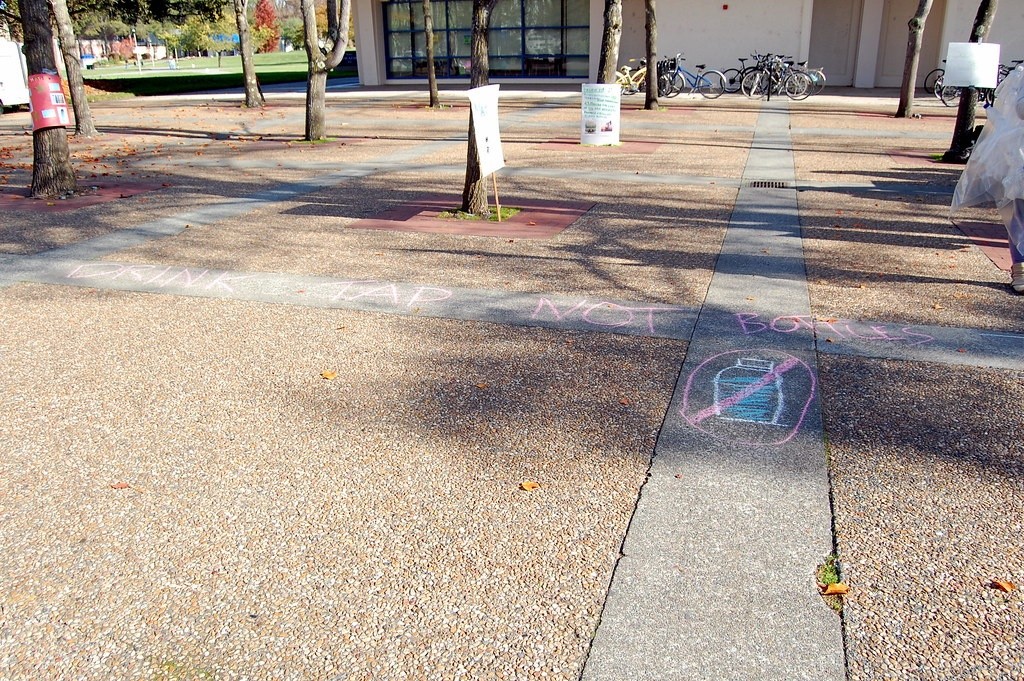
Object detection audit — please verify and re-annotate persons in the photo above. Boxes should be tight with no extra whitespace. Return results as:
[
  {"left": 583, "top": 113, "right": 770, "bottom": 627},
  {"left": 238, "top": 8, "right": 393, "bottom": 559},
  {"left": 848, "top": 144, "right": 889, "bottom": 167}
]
[
  {"left": 169, "top": 56, "right": 175, "bottom": 69},
  {"left": 1008, "top": 76, "right": 1024, "bottom": 292}
]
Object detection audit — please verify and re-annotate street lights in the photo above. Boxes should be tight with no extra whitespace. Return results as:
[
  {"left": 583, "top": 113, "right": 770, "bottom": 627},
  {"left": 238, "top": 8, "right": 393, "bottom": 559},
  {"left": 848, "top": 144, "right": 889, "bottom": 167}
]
[{"left": 131, "top": 28, "right": 142, "bottom": 71}]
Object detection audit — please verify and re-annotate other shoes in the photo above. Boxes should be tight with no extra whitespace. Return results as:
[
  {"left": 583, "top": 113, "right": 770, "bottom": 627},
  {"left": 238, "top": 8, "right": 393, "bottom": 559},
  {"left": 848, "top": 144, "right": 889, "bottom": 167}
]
[{"left": 1011, "top": 262, "right": 1024, "bottom": 296}]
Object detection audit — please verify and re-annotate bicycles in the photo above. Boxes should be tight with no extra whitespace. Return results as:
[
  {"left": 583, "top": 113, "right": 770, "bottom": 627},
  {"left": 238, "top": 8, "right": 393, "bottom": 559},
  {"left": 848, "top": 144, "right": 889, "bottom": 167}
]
[
  {"left": 612, "top": 51, "right": 677, "bottom": 96},
  {"left": 720, "top": 50, "right": 827, "bottom": 103},
  {"left": 657, "top": 53, "right": 727, "bottom": 100},
  {"left": 924, "top": 59, "right": 1024, "bottom": 107}
]
